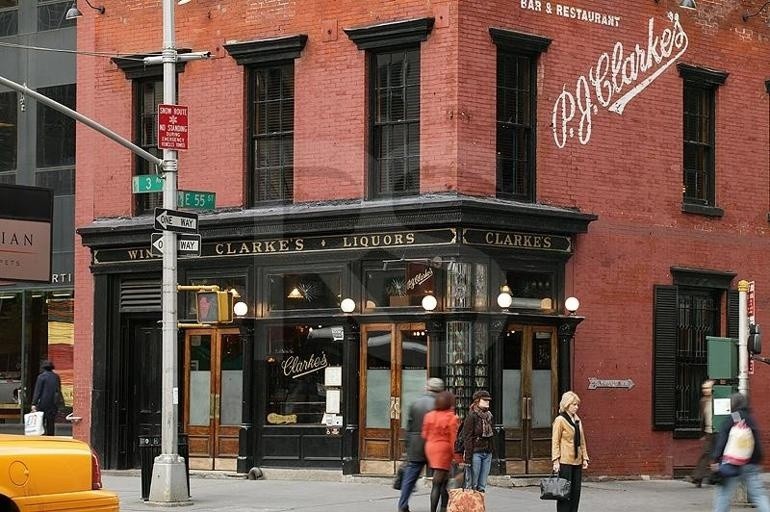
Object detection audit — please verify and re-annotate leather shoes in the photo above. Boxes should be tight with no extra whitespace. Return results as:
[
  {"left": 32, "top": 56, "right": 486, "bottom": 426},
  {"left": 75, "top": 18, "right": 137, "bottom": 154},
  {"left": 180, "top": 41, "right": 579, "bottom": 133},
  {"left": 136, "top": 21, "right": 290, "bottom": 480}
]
[{"left": 685, "top": 475, "right": 703, "bottom": 486}]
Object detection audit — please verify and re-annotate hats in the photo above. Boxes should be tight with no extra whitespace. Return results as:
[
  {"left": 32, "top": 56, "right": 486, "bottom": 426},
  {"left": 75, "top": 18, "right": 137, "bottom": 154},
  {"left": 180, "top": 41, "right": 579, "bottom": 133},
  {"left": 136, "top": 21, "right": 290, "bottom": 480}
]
[
  {"left": 427, "top": 378, "right": 445, "bottom": 392},
  {"left": 472, "top": 391, "right": 491, "bottom": 399}
]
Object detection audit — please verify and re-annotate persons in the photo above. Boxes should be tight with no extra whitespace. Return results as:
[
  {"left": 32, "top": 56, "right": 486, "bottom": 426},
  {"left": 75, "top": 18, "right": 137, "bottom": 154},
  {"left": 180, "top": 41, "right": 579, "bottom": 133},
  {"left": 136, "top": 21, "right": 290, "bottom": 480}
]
[
  {"left": 682, "top": 379, "right": 713, "bottom": 488},
  {"left": 550, "top": 391, "right": 590, "bottom": 512},
  {"left": 711, "top": 392, "right": 769, "bottom": 511},
  {"left": 420, "top": 390, "right": 459, "bottom": 511},
  {"left": 398, "top": 376, "right": 444, "bottom": 511},
  {"left": 463, "top": 389, "right": 493, "bottom": 493},
  {"left": 30, "top": 359, "right": 62, "bottom": 435}
]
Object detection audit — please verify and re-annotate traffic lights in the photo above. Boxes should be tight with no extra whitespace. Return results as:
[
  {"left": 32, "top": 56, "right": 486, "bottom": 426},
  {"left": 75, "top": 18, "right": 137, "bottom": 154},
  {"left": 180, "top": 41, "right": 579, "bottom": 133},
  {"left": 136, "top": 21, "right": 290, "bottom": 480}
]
[{"left": 195, "top": 290, "right": 223, "bottom": 326}]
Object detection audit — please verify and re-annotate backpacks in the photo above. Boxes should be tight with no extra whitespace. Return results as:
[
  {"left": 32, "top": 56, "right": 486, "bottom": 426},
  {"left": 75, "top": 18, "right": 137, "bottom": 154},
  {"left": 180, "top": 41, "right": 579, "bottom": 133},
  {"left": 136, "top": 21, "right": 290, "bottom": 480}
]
[
  {"left": 722, "top": 412, "right": 755, "bottom": 464},
  {"left": 453, "top": 416, "right": 476, "bottom": 454}
]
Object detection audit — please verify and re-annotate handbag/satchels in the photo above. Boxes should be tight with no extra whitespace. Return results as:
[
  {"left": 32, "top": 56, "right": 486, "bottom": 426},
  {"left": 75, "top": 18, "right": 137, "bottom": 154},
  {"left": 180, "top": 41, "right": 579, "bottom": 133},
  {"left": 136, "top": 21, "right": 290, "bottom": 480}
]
[
  {"left": 393, "top": 467, "right": 417, "bottom": 493},
  {"left": 24, "top": 412, "right": 45, "bottom": 434},
  {"left": 539, "top": 477, "right": 572, "bottom": 500},
  {"left": 447, "top": 489, "right": 486, "bottom": 511},
  {"left": 718, "top": 458, "right": 743, "bottom": 476},
  {"left": 54, "top": 391, "right": 66, "bottom": 409}
]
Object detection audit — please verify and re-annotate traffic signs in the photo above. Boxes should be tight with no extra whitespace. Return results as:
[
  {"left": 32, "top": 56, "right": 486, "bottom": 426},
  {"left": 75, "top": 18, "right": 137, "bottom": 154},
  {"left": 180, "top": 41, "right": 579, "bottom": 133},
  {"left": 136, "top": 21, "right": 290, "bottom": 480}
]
[
  {"left": 153, "top": 205, "right": 200, "bottom": 233},
  {"left": 149, "top": 232, "right": 203, "bottom": 259}
]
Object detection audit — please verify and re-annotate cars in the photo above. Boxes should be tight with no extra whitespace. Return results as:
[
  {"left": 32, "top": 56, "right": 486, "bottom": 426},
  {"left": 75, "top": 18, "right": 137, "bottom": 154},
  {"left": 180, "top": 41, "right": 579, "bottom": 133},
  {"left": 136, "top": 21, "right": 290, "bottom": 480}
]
[
  {"left": 269, "top": 384, "right": 323, "bottom": 425},
  {"left": 1, "top": 432, "right": 121, "bottom": 512}
]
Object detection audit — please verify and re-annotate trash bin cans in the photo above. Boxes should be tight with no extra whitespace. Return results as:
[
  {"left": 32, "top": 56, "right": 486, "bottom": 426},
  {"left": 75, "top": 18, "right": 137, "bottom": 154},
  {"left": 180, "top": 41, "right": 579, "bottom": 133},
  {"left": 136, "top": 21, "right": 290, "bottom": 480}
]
[{"left": 137, "top": 433, "right": 189, "bottom": 500}]
